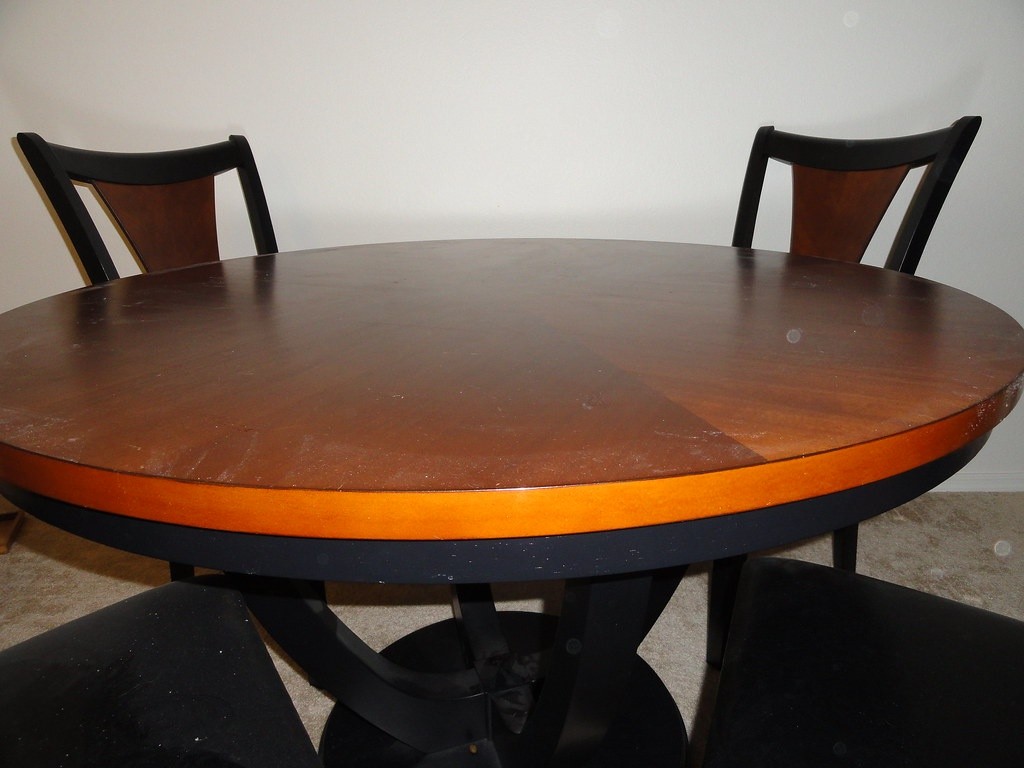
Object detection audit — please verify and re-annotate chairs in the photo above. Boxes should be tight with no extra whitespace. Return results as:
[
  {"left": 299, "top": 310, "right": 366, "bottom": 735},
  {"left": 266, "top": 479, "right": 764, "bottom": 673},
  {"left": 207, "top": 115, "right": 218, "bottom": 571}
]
[
  {"left": 703, "top": 558, "right": 1024, "bottom": 768},
  {"left": 17, "top": 131, "right": 279, "bottom": 580},
  {"left": 0, "top": 575, "right": 321, "bottom": 768},
  {"left": 733, "top": 118, "right": 981, "bottom": 570}
]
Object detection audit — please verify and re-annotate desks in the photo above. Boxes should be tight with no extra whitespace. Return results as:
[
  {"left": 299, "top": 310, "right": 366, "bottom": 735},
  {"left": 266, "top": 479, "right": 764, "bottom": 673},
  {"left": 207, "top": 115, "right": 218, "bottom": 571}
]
[{"left": 0, "top": 241, "right": 1024, "bottom": 768}]
